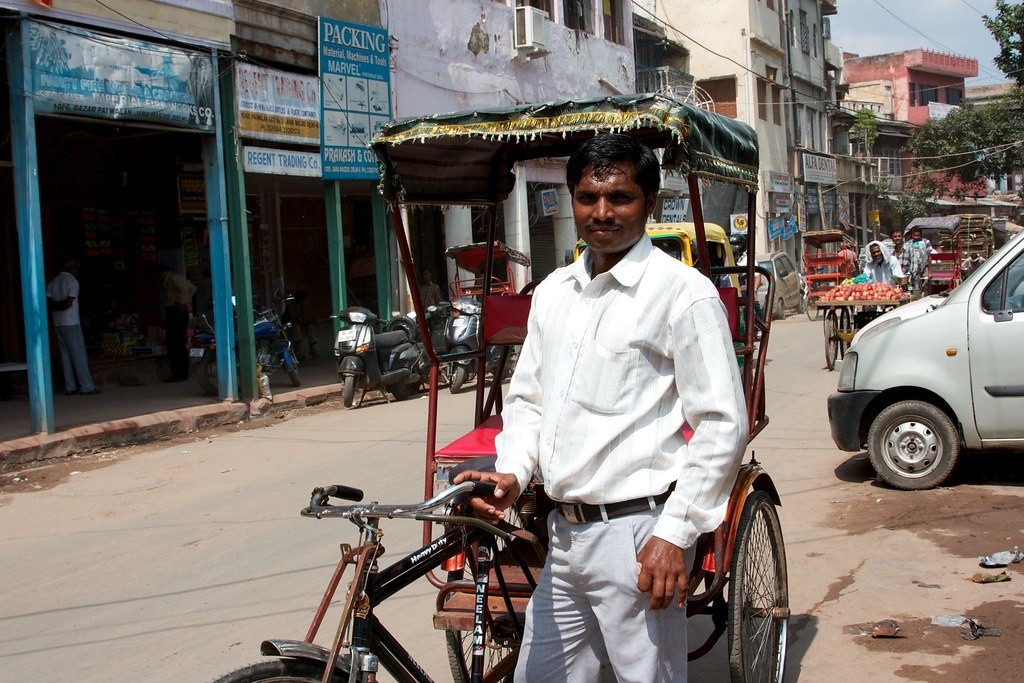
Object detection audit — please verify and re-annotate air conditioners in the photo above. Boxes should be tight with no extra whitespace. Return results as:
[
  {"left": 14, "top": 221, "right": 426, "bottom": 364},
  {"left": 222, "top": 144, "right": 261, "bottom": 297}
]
[{"left": 514, "top": 6, "right": 549, "bottom": 49}]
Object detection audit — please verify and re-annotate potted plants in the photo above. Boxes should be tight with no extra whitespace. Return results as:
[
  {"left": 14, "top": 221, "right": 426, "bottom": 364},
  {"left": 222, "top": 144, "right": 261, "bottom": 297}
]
[{"left": 849, "top": 107, "right": 880, "bottom": 163}]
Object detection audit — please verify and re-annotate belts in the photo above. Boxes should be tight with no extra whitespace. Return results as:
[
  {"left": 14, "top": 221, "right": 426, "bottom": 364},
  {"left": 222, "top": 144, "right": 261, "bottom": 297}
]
[{"left": 555, "top": 481, "right": 679, "bottom": 524}]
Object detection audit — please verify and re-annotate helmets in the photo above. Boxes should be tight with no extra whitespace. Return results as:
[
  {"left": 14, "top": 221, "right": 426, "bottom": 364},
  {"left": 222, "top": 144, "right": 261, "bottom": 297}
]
[
  {"left": 731, "top": 246, "right": 739, "bottom": 264},
  {"left": 729, "top": 233, "right": 746, "bottom": 253}
]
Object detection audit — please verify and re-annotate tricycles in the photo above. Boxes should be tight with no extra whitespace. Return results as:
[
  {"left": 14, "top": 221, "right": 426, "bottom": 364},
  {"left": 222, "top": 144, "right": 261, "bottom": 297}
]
[
  {"left": 903, "top": 216, "right": 963, "bottom": 295},
  {"left": 211, "top": 90, "right": 790, "bottom": 683},
  {"left": 800, "top": 231, "right": 863, "bottom": 322}
]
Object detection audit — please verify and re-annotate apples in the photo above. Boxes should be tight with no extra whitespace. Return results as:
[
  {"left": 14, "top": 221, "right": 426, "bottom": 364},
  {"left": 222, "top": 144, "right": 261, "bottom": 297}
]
[{"left": 819, "top": 282, "right": 909, "bottom": 301}]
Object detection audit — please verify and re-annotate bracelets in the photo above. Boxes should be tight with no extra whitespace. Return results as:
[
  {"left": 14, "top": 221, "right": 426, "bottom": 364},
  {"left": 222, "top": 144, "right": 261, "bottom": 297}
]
[{"left": 755, "top": 290, "right": 757, "bottom": 293}]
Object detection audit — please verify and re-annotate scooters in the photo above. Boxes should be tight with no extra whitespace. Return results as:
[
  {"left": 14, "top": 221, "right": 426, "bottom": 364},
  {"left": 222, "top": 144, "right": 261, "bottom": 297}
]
[
  {"left": 328, "top": 305, "right": 422, "bottom": 408},
  {"left": 396, "top": 305, "right": 453, "bottom": 385},
  {"left": 438, "top": 295, "right": 513, "bottom": 394}
]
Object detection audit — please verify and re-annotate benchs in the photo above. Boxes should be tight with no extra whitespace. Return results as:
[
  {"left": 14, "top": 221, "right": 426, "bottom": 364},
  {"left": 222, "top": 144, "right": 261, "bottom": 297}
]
[
  {"left": 806, "top": 256, "right": 845, "bottom": 281},
  {"left": 432, "top": 286, "right": 738, "bottom": 471},
  {"left": 930, "top": 252, "right": 959, "bottom": 278}
]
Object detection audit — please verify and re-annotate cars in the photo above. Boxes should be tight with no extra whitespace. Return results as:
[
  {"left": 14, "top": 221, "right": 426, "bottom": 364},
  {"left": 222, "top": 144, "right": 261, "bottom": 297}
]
[{"left": 826, "top": 228, "right": 1024, "bottom": 492}]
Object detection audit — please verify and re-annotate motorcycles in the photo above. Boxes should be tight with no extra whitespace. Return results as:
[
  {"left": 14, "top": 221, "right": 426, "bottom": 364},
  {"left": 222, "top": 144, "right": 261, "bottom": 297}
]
[{"left": 191, "top": 295, "right": 302, "bottom": 398}]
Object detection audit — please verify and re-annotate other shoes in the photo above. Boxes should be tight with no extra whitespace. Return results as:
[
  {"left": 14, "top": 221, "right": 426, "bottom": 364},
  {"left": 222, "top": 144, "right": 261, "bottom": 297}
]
[
  {"left": 79, "top": 388, "right": 101, "bottom": 394},
  {"left": 161, "top": 374, "right": 185, "bottom": 383},
  {"left": 65, "top": 388, "right": 80, "bottom": 394}
]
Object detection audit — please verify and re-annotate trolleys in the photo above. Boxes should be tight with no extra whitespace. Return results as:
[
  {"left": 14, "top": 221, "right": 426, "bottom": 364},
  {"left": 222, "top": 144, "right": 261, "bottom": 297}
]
[{"left": 813, "top": 294, "right": 914, "bottom": 373}]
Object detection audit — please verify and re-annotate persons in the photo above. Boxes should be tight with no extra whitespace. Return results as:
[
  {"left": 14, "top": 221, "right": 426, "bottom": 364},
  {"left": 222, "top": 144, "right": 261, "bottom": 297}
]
[
  {"left": 155, "top": 264, "right": 197, "bottom": 383},
  {"left": 283, "top": 289, "right": 321, "bottom": 365},
  {"left": 418, "top": 268, "right": 442, "bottom": 309},
  {"left": 904, "top": 227, "right": 932, "bottom": 296},
  {"left": 454, "top": 133, "right": 748, "bottom": 683},
  {"left": 863, "top": 240, "right": 905, "bottom": 313},
  {"left": 91, "top": 277, "right": 116, "bottom": 353},
  {"left": 45, "top": 262, "right": 96, "bottom": 395},
  {"left": 838, "top": 241, "right": 860, "bottom": 276},
  {"left": 717, "top": 233, "right": 764, "bottom": 337},
  {"left": 961, "top": 253, "right": 985, "bottom": 268},
  {"left": 891, "top": 229, "right": 920, "bottom": 300}
]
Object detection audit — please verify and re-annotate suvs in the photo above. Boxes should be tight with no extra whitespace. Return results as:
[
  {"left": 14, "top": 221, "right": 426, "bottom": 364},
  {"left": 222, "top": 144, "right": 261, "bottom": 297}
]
[{"left": 753, "top": 250, "right": 805, "bottom": 321}]
